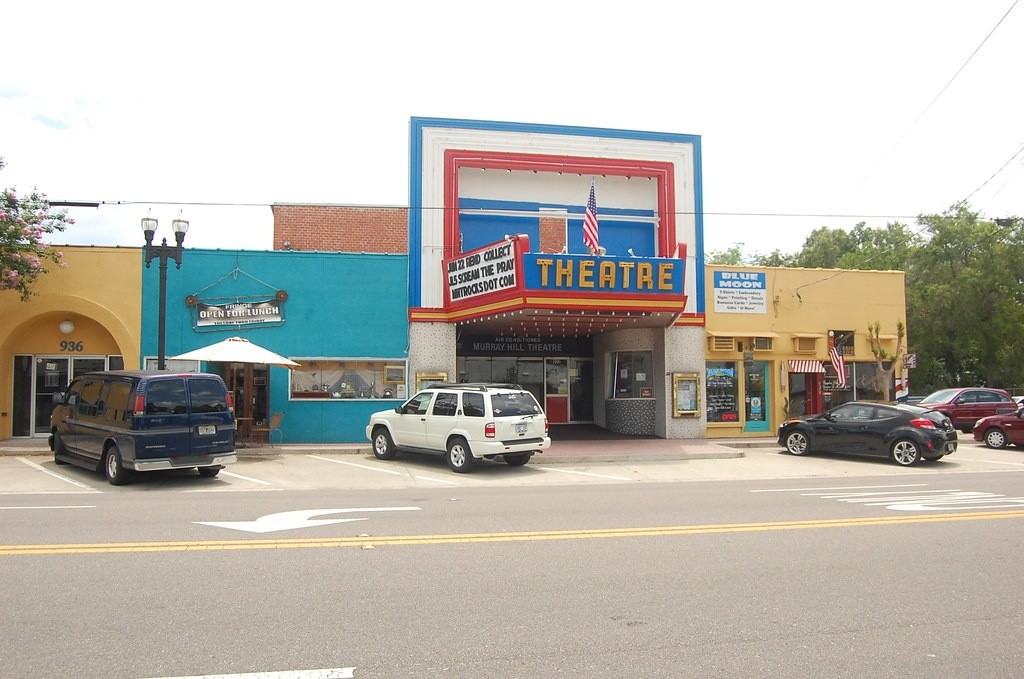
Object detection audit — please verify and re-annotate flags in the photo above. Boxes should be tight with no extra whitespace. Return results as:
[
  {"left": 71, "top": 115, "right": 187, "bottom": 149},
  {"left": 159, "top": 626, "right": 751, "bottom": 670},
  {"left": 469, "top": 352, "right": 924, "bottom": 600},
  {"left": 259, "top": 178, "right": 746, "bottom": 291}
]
[
  {"left": 829, "top": 349, "right": 846, "bottom": 388},
  {"left": 583, "top": 185, "right": 597, "bottom": 255}
]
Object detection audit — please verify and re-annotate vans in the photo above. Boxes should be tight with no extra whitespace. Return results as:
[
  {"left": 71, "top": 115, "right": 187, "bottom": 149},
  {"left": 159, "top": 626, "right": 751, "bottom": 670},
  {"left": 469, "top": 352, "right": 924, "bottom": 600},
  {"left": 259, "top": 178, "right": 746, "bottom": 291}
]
[{"left": 48, "top": 370, "right": 238, "bottom": 485}]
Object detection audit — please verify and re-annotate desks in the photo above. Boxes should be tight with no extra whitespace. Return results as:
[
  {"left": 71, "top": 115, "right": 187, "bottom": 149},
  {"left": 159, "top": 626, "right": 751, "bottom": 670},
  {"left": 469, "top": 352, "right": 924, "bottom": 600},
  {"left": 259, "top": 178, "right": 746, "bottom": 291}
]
[{"left": 233, "top": 417, "right": 255, "bottom": 448}]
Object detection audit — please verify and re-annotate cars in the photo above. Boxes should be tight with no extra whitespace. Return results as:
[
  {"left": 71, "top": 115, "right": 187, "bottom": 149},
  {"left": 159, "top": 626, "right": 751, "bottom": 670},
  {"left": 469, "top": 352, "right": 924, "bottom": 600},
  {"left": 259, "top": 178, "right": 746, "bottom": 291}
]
[
  {"left": 1011, "top": 396, "right": 1024, "bottom": 408},
  {"left": 972, "top": 406, "right": 1024, "bottom": 449},
  {"left": 777, "top": 400, "right": 957, "bottom": 467},
  {"left": 915, "top": 388, "right": 1018, "bottom": 433}
]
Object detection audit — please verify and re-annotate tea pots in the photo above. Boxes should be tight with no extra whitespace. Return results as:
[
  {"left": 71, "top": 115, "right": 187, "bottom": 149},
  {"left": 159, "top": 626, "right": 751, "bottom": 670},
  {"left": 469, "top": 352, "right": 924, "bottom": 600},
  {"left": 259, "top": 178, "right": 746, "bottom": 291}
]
[{"left": 331, "top": 392, "right": 342, "bottom": 398}]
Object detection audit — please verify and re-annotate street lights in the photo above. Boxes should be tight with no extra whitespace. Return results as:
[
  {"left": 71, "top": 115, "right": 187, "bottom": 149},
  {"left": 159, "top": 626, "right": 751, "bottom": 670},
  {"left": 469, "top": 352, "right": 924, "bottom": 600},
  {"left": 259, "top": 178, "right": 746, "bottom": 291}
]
[{"left": 141, "top": 207, "right": 189, "bottom": 370}]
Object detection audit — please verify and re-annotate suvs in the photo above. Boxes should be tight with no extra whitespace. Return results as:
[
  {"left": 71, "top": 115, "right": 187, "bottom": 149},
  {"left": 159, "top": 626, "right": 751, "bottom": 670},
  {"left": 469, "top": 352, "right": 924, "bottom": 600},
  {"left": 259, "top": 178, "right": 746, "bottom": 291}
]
[{"left": 366, "top": 382, "right": 551, "bottom": 473}]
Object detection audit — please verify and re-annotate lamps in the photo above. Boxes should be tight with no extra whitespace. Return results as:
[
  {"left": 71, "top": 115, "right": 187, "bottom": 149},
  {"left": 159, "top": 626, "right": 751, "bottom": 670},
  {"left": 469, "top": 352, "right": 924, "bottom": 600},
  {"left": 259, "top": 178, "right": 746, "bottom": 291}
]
[
  {"left": 60, "top": 320, "right": 75, "bottom": 334},
  {"left": 559, "top": 245, "right": 567, "bottom": 254},
  {"left": 628, "top": 248, "right": 635, "bottom": 256}
]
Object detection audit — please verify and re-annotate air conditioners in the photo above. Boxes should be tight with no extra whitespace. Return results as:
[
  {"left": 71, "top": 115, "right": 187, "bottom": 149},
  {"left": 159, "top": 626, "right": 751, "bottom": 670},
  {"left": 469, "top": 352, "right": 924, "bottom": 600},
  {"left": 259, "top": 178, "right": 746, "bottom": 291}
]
[
  {"left": 749, "top": 337, "right": 773, "bottom": 352},
  {"left": 707, "top": 337, "right": 735, "bottom": 352},
  {"left": 792, "top": 338, "right": 817, "bottom": 355}
]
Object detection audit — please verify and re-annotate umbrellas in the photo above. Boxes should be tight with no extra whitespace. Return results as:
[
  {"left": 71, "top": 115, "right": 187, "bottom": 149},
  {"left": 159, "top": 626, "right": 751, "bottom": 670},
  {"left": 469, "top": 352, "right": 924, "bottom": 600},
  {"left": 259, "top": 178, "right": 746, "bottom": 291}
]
[{"left": 169, "top": 336, "right": 301, "bottom": 418}]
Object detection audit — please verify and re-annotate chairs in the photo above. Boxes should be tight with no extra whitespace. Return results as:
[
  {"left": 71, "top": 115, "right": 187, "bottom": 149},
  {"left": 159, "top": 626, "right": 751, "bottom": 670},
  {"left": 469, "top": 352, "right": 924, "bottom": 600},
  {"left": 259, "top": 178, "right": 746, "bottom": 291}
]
[{"left": 250, "top": 412, "right": 285, "bottom": 447}]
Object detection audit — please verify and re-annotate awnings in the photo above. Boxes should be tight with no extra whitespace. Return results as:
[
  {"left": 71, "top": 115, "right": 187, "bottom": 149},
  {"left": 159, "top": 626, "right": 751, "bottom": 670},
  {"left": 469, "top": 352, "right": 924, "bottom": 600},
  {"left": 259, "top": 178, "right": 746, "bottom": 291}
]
[{"left": 790, "top": 359, "right": 826, "bottom": 375}]
